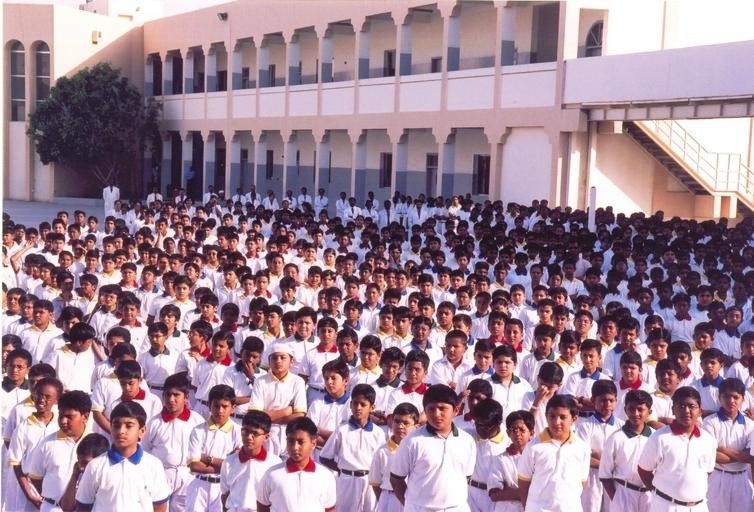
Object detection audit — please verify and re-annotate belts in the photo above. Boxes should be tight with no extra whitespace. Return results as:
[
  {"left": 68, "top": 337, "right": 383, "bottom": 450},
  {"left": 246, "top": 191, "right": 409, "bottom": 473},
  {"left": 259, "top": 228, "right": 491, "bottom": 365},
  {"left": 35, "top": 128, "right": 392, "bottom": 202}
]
[
  {"left": 197, "top": 399, "right": 209, "bottom": 406},
  {"left": 196, "top": 474, "right": 220, "bottom": 482},
  {"left": 148, "top": 385, "right": 164, "bottom": 390},
  {"left": 342, "top": 469, "right": 369, "bottom": 476},
  {"left": 310, "top": 386, "right": 326, "bottom": 392},
  {"left": 42, "top": 497, "right": 59, "bottom": 506},
  {"left": 468, "top": 479, "right": 487, "bottom": 489},
  {"left": 614, "top": 478, "right": 703, "bottom": 506},
  {"left": 230, "top": 413, "right": 245, "bottom": 418},
  {"left": 579, "top": 412, "right": 593, "bottom": 417},
  {"left": 714, "top": 467, "right": 746, "bottom": 473}
]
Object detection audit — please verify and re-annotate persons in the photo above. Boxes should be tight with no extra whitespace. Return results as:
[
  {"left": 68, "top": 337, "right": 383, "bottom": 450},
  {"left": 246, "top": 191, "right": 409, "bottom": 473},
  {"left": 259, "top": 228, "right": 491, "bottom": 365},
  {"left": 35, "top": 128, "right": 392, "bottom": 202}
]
[
  {"left": 184, "top": 166, "right": 197, "bottom": 198},
  {"left": 101, "top": 180, "right": 120, "bottom": 218},
  {"left": 0, "top": 184, "right": 753, "bottom": 512}
]
